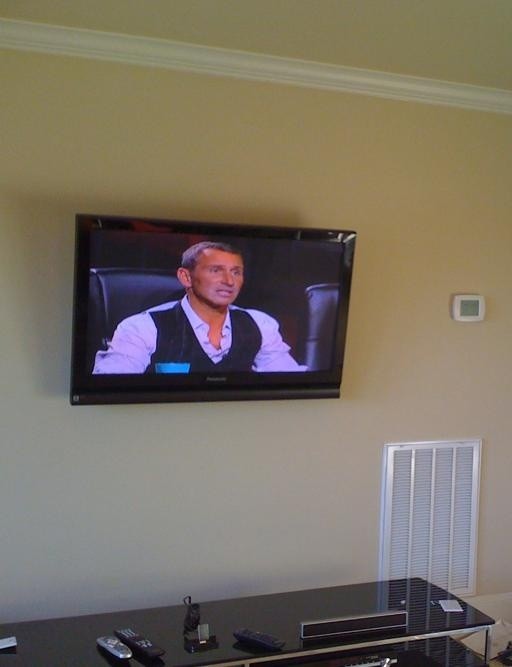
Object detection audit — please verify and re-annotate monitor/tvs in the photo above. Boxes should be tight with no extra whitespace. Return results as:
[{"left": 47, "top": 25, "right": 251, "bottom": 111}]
[{"left": 70, "top": 214, "right": 355, "bottom": 405}]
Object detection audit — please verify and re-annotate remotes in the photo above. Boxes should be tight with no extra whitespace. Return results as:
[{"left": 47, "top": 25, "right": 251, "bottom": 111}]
[
  {"left": 233, "top": 628, "right": 285, "bottom": 652},
  {"left": 96, "top": 635, "right": 133, "bottom": 660},
  {"left": 114, "top": 628, "right": 166, "bottom": 657}
]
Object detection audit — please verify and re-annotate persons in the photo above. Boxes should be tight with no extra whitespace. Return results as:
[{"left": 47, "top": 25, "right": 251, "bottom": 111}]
[{"left": 92, "top": 240, "right": 308, "bottom": 373}]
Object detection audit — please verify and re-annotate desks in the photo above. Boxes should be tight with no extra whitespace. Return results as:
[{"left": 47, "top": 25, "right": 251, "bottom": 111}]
[{"left": 1, "top": 574, "right": 499, "bottom": 667}]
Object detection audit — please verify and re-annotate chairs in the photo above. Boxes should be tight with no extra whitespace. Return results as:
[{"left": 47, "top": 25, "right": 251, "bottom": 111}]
[
  {"left": 84, "top": 268, "right": 190, "bottom": 370},
  {"left": 289, "top": 284, "right": 338, "bottom": 377}
]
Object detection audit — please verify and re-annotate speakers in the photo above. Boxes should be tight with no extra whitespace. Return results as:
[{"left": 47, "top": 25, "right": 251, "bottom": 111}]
[{"left": 301, "top": 611, "right": 409, "bottom": 639}]
